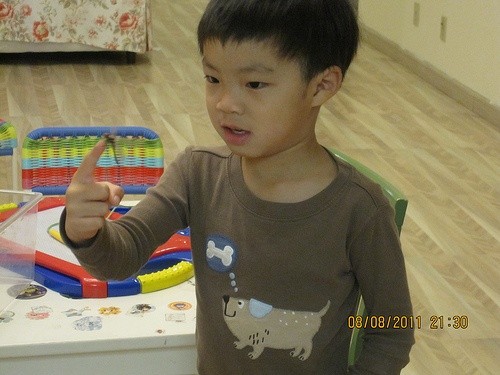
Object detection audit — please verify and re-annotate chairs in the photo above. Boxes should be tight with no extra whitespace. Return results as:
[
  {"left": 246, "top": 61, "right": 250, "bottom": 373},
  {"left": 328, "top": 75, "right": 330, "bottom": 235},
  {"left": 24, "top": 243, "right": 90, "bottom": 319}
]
[
  {"left": 331, "top": 146, "right": 409, "bottom": 375},
  {"left": 20, "top": 126, "right": 166, "bottom": 193},
  {"left": 0, "top": 118, "right": 22, "bottom": 193}
]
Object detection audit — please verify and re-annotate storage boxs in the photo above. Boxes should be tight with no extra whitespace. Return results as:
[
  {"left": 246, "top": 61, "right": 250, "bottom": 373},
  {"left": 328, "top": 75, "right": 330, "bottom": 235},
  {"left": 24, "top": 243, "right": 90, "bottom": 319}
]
[{"left": 1, "top": 189, "right": 48, "bottom": 318}]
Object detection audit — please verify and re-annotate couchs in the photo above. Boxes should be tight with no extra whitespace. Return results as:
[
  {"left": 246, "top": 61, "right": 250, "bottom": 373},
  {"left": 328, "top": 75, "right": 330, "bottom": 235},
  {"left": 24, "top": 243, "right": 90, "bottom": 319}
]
[{"left": 0, "top": 1, "right": 151, "bottom": 62}]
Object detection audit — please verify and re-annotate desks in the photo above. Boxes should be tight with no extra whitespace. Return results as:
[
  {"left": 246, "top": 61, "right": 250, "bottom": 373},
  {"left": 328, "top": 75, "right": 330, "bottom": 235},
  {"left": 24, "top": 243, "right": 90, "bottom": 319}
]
[{"left": 0, "top": 200, "right": 201, "bottom": 375}]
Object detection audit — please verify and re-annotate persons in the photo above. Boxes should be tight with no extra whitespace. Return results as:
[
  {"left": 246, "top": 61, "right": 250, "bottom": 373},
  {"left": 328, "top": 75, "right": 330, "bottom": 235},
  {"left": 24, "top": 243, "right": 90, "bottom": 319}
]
[{"left": 59, "top": 0, "right": 416, "bottom": 374}]
